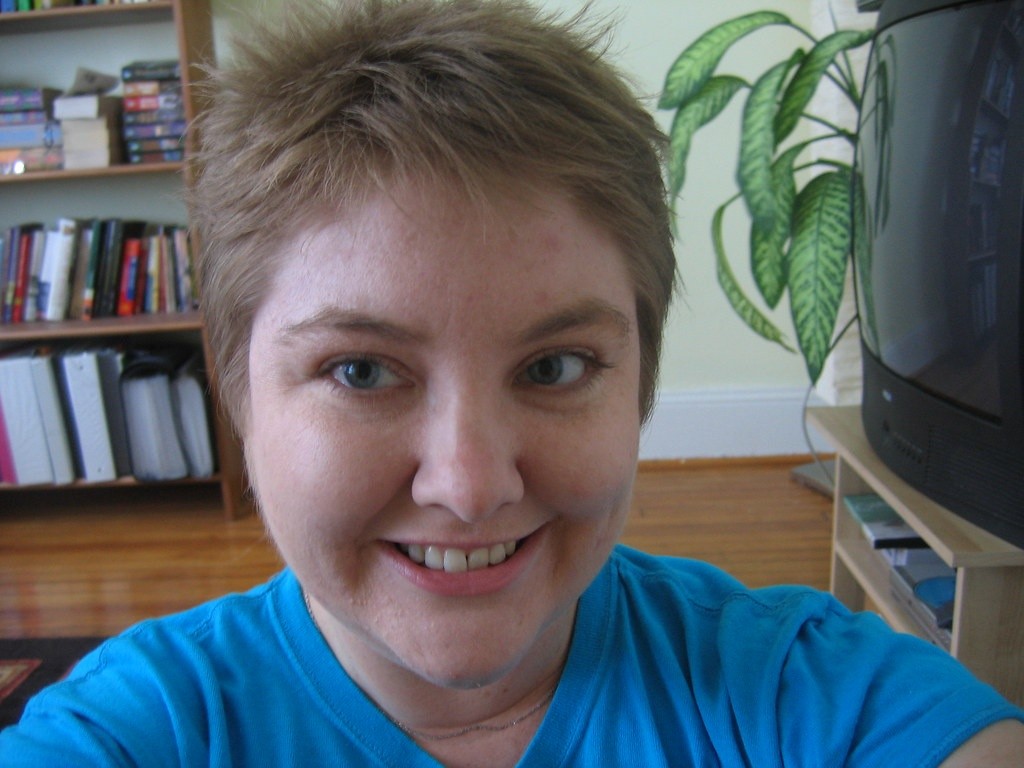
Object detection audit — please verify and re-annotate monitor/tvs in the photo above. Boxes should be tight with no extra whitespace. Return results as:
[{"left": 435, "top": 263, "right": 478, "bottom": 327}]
[{"left": 854, "top": 0, "right": 1024, "bottom": 553}]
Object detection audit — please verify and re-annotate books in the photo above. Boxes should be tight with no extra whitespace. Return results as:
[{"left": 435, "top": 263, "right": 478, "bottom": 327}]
[
  {"left": 0, "top": 59, "right": 189, "bottom": 174},
  {"left": 0, "top": 342, "right": 216, "bottom": 489},
  {"left": 0, "top": 217, "right": 196, "bottom": 324},
  {"left": 0, "top": 0, "right": 152, "bottom": 13}
]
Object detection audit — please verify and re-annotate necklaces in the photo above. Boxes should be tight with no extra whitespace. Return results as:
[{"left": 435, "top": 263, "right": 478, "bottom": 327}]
[{"left": 304, "top": 587, "right": 558, "bottom": 740}]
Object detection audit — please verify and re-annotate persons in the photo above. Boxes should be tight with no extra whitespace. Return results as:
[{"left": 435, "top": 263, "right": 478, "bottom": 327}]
[{"left": 0, "top": 0, "right": 1022, "bottom": 768}]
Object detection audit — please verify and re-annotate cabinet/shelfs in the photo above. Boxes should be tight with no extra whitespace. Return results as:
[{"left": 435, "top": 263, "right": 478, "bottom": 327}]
[
  {"left": 806, "top": 402, "right": 1024, "bottom": 710},
  {"left": 0, "top": 0, "right": 246, "bottom": 523}
]
